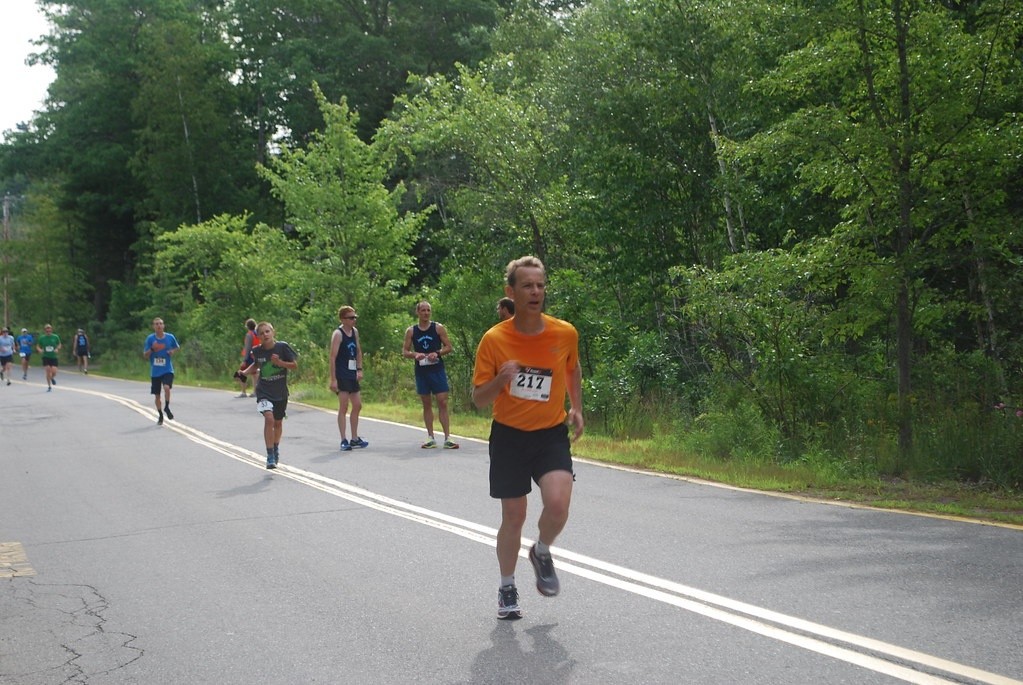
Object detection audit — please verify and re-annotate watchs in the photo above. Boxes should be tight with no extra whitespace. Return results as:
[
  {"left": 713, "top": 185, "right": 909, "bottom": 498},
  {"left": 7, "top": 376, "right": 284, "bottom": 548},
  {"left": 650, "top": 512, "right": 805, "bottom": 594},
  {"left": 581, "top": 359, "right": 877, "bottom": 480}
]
[{"left": 436, "top": 349, "right": 441, "bottom": 358}]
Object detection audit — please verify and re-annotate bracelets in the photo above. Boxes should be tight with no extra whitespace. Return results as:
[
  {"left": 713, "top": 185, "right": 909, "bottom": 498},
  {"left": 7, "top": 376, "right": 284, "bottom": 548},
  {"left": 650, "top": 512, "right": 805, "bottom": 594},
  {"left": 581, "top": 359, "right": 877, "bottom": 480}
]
[
  {"left": 27, "top": 342, "right": 28, "bottom": 344},
  {"left": 57, "top": 348, "right": 59, "bottom": 350},
  {"left": 357, "top": 368, "right": 362, "bottom": 370},
  {"left": 150, "top": 347, "right": 155, "bottom": 353}
]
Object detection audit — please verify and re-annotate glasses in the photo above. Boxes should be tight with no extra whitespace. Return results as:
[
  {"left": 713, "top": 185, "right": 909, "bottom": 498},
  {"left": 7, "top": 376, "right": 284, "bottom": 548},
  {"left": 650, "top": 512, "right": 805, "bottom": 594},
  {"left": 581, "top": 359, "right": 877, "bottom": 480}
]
[{"left": 343, "top": 315, "right": 357, "bottom": 320}]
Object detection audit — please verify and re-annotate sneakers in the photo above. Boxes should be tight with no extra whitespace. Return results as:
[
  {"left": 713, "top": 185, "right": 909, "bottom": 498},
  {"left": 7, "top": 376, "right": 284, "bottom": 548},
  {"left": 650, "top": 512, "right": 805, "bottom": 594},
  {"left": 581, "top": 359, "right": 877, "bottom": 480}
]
[
  {"left": 266, "top": 456, "right": 276, "bottom": 469},
  {"left": 529, "top": 543, "right": 560, "bottom": 597},
  {"left": 422, "top": 436, "right": 437, "bottom": 448},
  {"left": 275, "top": 447, "right": 279, "bottom": 464},
  {"left": 497, "top": 584, "right": 521, "bottom": 618},
  {"left": 443, "top": 436, "right": 459, "bottom": 448},
  {"left": 350, "top": 437, "right": 368, "bottom": 448},
  {"left": 340, "top": 439, "right": 352, "bottom": 450}
]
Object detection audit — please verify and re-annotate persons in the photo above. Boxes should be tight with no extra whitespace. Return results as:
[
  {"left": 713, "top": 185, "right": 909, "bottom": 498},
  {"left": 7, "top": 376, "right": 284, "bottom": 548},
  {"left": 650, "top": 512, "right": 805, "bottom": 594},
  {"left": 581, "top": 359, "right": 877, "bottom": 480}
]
[
  {"left": 496, "top": 297, "right": 514, "bottom": 322},
  {"left": 72, "top": 328, "right": 91, "bottom": 375},
  {"left": 234, "top": 318, "right": 261, "bottom": 398},
  {"left": 470, "top": 255, "right": 586, "bottom": 618},
  {"left": 237, "top": 321, "right": 298, "bottom": 469},
  {"left": 403, "top": 300, "right": 459, "bottom": 449},
  {"left": 329, "top": 305, "right": 369, "bottom": 451},
  {"left": 15, "top": 328, "right": 34, "bottom": 379},
  {"left": 35, "top": 323, "right": 62, "bottom": 392},
  {"left": 143, "top": 317, "right": 180, "bottom": 425},
  {"left": 0, "top": 327, "right": 18, "bottom": 385}
]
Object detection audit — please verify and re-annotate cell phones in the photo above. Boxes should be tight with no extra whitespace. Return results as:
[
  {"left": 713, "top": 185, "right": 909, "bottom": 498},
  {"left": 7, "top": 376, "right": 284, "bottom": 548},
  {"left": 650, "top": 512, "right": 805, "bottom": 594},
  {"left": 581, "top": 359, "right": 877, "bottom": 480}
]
[{"left": 234, "top": 372, "right": 248, "bottom": 384}]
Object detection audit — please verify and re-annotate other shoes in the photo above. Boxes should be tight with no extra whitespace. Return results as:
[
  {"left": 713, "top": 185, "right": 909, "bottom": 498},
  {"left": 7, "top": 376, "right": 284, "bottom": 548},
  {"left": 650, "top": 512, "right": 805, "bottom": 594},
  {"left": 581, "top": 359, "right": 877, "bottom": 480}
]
[
  {"left": 164, "top": 408, "right": 173, "bottom": 419},
  {"left": 51, "top": 377, "right": 56, "bottom": 385},
  {"left": 158, "top": 416, "right": 163, "bottom": 425}
]
